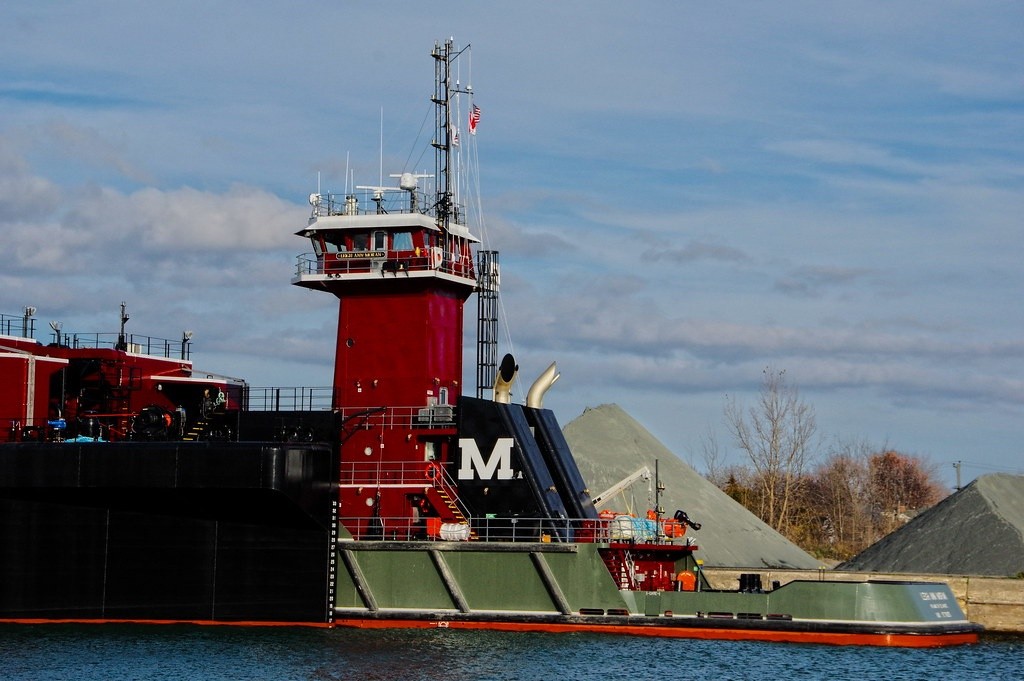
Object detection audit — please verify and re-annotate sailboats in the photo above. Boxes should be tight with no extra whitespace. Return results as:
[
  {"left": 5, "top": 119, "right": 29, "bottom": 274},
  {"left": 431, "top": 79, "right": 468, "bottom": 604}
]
[{"left": 1, "top": 36, "right": 979, "bottom": 647}]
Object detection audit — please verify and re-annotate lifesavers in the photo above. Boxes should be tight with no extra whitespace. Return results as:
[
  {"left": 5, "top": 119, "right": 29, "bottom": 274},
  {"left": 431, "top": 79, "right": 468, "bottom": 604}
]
[
  {"left": 664, "top": 518, "right": 687, "bottom": 537},
  {"left": 425, "top": 462, "right": 441, "bottom": 481},
  {"left": 677, "top": 570, "right": 695, "bottom": 590}
]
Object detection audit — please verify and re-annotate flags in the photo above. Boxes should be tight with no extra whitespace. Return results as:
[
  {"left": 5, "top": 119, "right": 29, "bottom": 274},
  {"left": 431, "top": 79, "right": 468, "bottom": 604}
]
[{"left": 469, "top": 105, "right": 480, "bottom": 132}]
